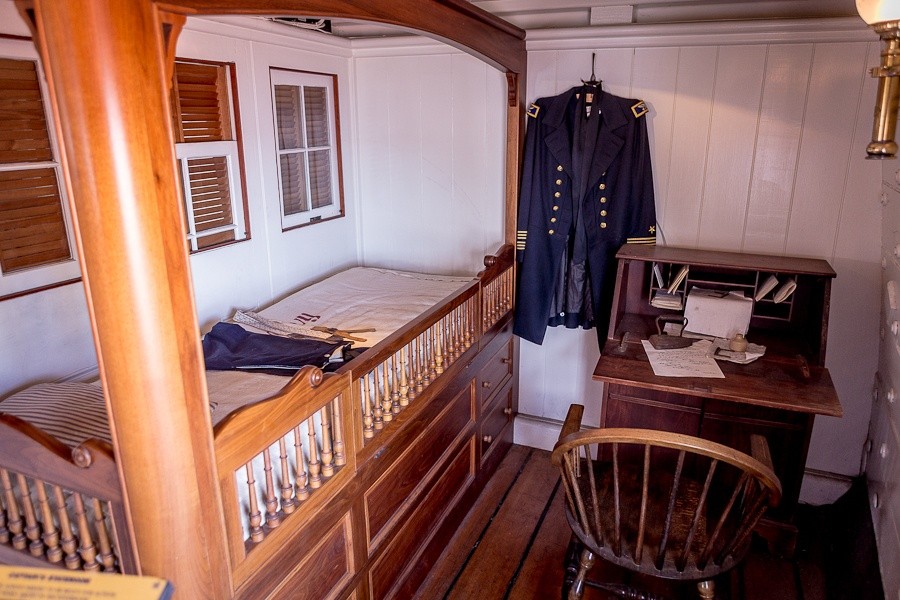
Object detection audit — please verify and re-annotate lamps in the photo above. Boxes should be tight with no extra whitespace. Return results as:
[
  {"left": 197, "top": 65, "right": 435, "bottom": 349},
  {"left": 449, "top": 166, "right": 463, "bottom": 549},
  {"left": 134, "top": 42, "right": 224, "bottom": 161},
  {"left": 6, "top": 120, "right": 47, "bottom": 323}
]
[{"left": 856, "top": 0, "right": 900, "bottom": 160}]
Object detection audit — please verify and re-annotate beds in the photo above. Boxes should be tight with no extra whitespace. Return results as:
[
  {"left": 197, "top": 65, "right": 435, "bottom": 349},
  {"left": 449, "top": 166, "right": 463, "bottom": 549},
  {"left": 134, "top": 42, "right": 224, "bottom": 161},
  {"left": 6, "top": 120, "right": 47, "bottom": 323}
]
[{"left": 0, "top": 244, "right": 518, "bottom": 581}]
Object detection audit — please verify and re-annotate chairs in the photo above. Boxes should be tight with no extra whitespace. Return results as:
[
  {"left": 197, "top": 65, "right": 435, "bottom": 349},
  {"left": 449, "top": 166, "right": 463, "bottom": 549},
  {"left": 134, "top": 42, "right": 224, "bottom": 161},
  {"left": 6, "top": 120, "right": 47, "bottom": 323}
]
[{"left": 552, "top": 404, "right": 783, "bottom": 600}]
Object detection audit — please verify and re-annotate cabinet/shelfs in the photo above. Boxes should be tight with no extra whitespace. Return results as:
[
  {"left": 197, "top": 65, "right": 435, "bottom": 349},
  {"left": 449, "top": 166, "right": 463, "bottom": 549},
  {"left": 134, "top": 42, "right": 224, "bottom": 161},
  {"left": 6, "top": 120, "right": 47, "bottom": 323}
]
[
  {"left": 592, "top": 241, "right": 844, "bottom": 515},
  {"left": 236, "top": 313, "right": 515, "bottom": 600}
]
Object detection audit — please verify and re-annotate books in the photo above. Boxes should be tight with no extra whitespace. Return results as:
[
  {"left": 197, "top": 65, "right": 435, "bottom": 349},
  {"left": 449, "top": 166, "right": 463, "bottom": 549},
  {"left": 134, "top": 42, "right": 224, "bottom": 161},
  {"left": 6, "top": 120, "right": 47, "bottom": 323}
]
[{"left": 667, "top": 265, "right": 689, "bottom": 295}]
